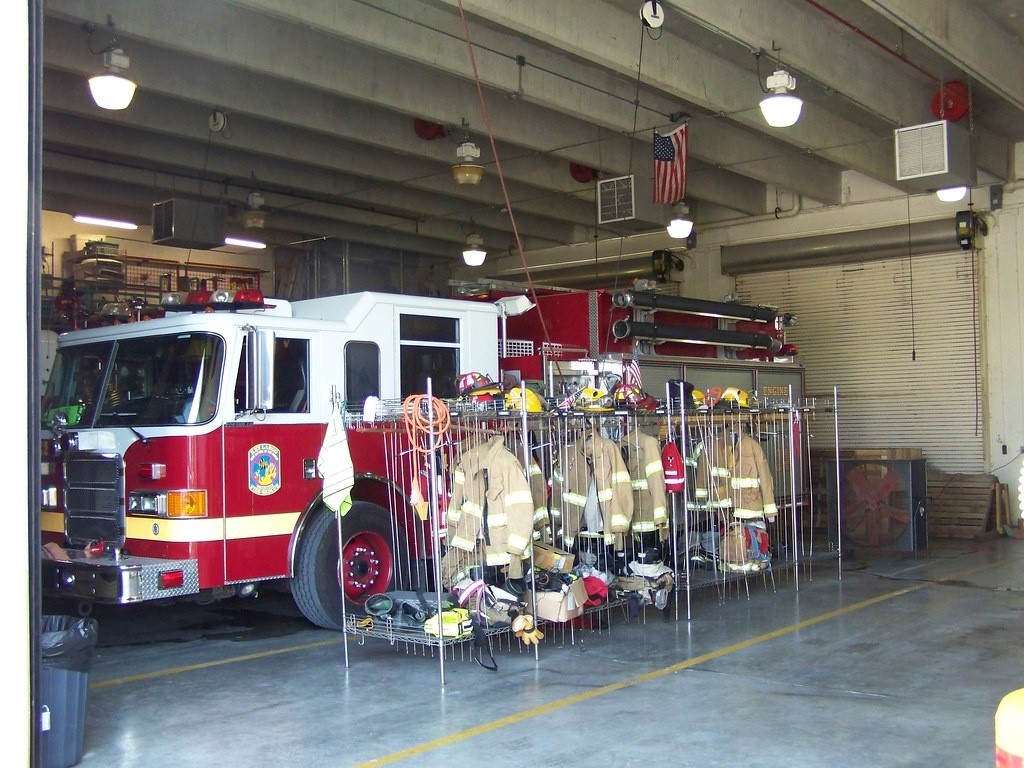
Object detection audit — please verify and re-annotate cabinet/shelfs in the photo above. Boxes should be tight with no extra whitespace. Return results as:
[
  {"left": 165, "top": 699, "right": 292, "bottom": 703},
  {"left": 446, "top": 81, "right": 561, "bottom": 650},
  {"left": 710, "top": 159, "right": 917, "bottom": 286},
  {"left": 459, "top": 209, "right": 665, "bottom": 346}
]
[{"left": 77, "top": 253, "right": 265, "bottom": 293}]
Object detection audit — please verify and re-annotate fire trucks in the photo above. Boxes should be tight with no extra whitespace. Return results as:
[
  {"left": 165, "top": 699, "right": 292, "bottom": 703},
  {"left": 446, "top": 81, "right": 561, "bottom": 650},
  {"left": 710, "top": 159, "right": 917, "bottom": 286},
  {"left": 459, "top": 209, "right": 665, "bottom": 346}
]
[{"left": 40, "top": 279, "right": 809, "bottom": 629}]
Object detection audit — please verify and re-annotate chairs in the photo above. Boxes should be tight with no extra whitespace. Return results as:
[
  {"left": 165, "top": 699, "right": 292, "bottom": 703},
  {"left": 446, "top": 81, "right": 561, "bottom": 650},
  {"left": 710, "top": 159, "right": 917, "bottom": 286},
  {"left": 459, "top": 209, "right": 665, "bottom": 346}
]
[{"left": 272, "top": 349, "right": 306, "bottom": 414}]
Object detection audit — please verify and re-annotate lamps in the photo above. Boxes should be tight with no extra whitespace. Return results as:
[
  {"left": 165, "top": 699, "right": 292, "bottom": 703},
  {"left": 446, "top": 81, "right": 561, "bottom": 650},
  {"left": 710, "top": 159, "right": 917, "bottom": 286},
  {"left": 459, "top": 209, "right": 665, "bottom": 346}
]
[
  {"left": 225, "top": 191, "right": 268, "bottom": 250},
  {"left": 753, "top": 39, "right": 804, "bottom": 128},
  {"left": 666, "top": 201, "right": 693, "bottom": 239},
  {"left": 82, "top": 14, "right": 138, "bottom": 111},
  {"left": 458, "top": 216, "right": 486, "bottom": 266},
  {"left": 447, "top": 117, "right": 485, "bottom": 185},
  {"left": 937, "top": 186, "right": 967, "bottom": 202}
]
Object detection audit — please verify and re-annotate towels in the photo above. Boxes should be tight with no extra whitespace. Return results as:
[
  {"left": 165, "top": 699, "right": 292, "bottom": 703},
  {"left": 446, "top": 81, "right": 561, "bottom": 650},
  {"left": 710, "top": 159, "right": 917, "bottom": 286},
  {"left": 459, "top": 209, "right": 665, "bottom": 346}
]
[{"left": 316, "top": 405, "right": 354, "bottom": 517}]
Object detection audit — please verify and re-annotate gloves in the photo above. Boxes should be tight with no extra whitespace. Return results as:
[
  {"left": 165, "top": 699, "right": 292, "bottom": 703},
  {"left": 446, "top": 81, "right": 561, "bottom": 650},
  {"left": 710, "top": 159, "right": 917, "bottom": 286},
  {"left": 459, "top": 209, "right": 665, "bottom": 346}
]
[{"left": 512, "top": 615, "right": 544, "bottom": 644}]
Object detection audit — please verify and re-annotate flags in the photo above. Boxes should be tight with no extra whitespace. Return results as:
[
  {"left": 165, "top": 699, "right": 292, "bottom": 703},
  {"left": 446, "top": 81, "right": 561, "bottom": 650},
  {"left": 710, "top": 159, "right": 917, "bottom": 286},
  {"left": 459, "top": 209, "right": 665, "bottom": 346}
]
[{"left": 653, "top": 124, "right": 689, "bottom": 206}]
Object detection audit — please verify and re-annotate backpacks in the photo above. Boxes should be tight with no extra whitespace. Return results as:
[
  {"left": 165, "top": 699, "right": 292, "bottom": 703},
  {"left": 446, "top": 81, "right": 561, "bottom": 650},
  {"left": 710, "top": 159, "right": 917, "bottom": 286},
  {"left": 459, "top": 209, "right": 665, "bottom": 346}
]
[{"left": 480, "top": 585, "right": 529, "bottom": 629}]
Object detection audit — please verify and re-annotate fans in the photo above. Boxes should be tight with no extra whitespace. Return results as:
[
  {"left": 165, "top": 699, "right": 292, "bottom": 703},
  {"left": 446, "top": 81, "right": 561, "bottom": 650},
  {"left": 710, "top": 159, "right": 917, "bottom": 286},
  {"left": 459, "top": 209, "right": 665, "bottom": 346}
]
[{"left": 825, "top": 456, "right": 928, "bottom": 556}]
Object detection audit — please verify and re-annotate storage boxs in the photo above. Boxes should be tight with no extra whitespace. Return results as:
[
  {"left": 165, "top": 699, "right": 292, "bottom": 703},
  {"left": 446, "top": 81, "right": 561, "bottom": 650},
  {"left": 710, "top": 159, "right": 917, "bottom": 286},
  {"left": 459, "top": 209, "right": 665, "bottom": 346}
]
[
  {"left": 524, "top": 578, "right": 589, "bottom": 623},
  {"left": 526, "top": 541, "right": 575, "bottom": 575},
  {"left": 720, "top": 527, "right": 751, "bottom": 562}
]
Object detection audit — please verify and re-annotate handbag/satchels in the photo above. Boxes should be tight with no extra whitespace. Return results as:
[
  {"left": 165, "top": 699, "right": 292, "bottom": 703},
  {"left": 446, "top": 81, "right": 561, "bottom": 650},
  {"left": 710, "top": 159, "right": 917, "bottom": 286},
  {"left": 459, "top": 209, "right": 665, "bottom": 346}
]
[{"left": 424, "top": 608, "right": 498, "bottom": 671}]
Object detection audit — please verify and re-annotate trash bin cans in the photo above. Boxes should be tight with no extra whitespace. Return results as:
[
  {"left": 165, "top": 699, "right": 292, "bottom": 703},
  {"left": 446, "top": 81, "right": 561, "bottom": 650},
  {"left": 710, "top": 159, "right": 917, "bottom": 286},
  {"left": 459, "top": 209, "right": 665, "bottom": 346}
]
[{"left": 36, "top": 612, "right": 99, "bottom": 768}]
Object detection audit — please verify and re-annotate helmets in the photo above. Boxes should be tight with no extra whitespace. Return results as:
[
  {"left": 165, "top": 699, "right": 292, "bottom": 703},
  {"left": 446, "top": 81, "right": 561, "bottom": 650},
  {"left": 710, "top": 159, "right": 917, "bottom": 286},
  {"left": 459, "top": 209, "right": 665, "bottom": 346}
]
[
  {"left": 721, "top": 387, "right": 750, "bottom": 407},
  {"left": 459, "top": 372, "right": 502, "bottom": 397},
  {"left": 613, "top": 385, "right": 658, "bottom": 410},
  {"left": 692, "top": 390, "right": 705, "bottom": 407},
  {"left": 504, "top": 387, "right": 544, "bottom": 412},
  {"left": 575, "top": 388, "right": 615, "bottom": 412},
  {"left": 466, "top": 393, "right": 495, "bottom": 402}
]
